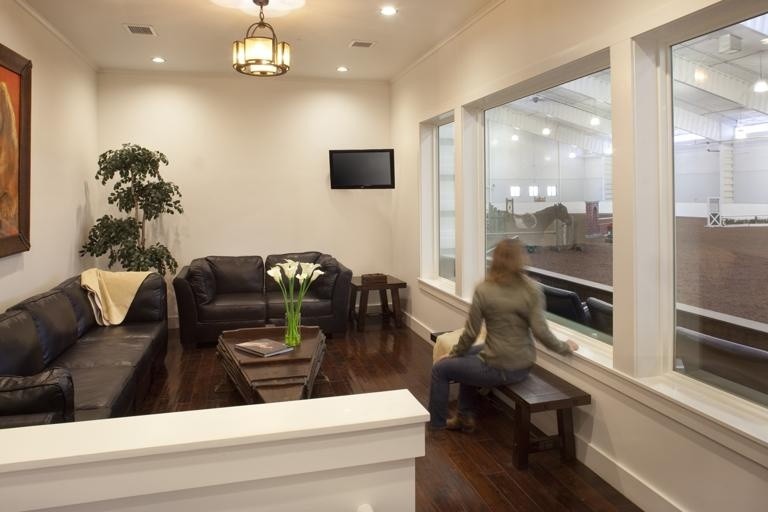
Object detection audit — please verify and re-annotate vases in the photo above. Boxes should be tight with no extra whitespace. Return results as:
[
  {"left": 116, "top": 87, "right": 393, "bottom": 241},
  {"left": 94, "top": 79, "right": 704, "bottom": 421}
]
[{"left": 284, "top": 312, "right": 302, "bottom": 346}]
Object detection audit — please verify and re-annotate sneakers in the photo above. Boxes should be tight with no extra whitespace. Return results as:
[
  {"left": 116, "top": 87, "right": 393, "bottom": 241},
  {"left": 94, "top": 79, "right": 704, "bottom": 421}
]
[{"left": 446, "top": 414, "right": 474, "bottom": 429}]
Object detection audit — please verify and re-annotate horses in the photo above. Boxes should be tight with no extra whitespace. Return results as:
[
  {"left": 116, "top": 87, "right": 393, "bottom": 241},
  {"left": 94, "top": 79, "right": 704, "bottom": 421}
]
[{"left": 498, "top": 202, "right": 573, "bottom": 267}]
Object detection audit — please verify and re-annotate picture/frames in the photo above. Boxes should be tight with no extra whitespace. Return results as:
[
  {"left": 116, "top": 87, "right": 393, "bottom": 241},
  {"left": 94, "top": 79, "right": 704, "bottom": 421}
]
[{"left": 0, "top": 44, "right": 31, "bottom": 258}]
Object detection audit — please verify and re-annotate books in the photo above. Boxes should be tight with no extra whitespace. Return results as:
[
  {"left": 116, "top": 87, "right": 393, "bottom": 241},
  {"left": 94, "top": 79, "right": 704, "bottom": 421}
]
[{"left": 235, "top": 338, "right": 293, "bottom": 358}]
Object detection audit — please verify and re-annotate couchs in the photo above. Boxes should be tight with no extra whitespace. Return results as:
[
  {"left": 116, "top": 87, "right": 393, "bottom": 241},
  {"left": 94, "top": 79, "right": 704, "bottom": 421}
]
[
  {"left": 0, "top": 268, "right": 167, "bottom": 430},
  {"left": 173, "top": 251, "right": 352, "bottom": 350}
]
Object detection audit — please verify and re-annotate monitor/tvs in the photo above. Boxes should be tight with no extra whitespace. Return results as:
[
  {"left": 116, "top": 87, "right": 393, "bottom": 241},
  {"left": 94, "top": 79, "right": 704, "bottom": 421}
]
[{"left": 329, "top": 150, "right": 395, "bottom": 190}]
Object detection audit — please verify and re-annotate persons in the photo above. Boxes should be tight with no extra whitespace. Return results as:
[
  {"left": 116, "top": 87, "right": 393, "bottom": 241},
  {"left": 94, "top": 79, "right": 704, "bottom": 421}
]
[{"left": 424, "top": 239, "right": 578, "bottom": 437}]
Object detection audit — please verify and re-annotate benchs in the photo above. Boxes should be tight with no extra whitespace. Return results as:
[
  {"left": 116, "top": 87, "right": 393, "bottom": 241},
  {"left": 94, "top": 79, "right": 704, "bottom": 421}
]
[{"left": 431, "top": 331, "right": 591, "bottom": 469}]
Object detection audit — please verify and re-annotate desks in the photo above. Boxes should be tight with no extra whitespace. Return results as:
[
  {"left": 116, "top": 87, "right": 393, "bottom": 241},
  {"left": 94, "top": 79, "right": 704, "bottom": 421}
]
[{"left": 351, "top": 274, "right": 407, "bottom": 331}]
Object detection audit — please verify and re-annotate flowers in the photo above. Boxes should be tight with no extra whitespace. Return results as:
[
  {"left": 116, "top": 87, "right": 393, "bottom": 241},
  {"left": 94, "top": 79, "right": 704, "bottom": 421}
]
[{"left": 267, "top": 258, "right": 325, "bottom": 313}]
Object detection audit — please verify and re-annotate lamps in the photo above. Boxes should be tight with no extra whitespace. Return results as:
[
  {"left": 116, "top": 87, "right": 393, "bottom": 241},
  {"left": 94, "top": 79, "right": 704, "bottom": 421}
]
[{"left": 232, "top": 0, "right": 291, "bottom": 77}]
